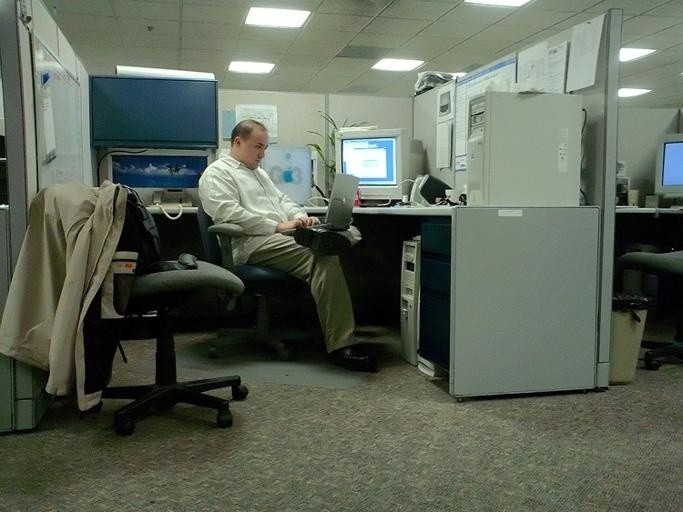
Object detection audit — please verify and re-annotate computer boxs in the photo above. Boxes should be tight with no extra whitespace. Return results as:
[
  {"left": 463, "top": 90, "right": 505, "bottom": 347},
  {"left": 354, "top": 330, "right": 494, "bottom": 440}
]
[
  {"left": 399, "top": 240, "right": 421, "bottom": 367},
  {"left": 465, "top": 91, "right": 584, "bottom": 207}
]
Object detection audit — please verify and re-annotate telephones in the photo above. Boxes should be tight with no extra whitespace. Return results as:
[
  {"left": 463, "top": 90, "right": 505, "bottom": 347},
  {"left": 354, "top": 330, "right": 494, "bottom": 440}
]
[{"left": 152, "top": 188, "right": 193, "bottom": 207}]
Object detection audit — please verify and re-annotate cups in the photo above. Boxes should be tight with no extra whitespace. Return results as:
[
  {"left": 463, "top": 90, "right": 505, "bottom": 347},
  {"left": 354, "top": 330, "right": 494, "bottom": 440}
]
[{"left": 630, "top": 189, "right": 640, "bottom": 208}]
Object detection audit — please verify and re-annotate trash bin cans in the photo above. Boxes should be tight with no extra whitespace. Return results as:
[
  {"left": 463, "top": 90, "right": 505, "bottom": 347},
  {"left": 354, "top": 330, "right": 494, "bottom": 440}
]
[{"left": 609, "top": 294, "right": 649, "bottom": 385}]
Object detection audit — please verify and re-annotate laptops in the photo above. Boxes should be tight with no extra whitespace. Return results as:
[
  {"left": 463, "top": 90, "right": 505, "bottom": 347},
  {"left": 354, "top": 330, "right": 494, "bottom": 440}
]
[{"left": 282, "top": 173, "right": 359, "bottom": 236}]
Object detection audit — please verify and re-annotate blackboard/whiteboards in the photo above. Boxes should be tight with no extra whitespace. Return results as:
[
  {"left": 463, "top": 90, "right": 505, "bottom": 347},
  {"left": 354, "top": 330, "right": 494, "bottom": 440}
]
[{"left": 219, "top": 146, "right": 313, "bottom": 207}]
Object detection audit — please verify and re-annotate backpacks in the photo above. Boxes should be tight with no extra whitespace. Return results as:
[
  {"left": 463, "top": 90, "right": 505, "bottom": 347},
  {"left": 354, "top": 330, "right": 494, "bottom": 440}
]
[{"left": 110, "top": 184, "right": 161, "bottom": 274}]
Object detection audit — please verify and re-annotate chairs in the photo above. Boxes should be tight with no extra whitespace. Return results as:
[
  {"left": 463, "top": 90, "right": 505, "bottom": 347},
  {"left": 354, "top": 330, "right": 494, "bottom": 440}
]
[
  {"left": 93, "top": 190, "right": 249, "bottom": 434},
  {"left": 196, "top": 202, "right": 303, "bottom": 361},
  {"left": 617, "top": 251, "right": 683, "bottom": 370}
]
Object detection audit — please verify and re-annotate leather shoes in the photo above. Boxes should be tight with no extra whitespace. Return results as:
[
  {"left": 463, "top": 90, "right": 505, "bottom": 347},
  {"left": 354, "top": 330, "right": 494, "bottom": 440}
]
[
  {"left": 294, "top": 227, "right": 351, "bottom": 255},
  {"left": 329, "top": 344, "right": 377, "bottom": 371}
]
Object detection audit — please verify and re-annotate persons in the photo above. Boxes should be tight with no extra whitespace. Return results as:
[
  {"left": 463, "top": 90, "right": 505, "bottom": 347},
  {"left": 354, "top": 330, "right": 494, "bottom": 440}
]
[{"left": 196, "top": 118, "right": 382, "bottom": 374}]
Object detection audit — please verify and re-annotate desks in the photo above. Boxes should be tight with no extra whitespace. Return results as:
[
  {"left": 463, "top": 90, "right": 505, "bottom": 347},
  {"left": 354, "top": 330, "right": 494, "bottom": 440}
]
[
  {"left": 616, "top": 207, "right": 683, "bottom": 214},
  {"left": 141, "top": 204, "right": 601, "bottom": 403}
]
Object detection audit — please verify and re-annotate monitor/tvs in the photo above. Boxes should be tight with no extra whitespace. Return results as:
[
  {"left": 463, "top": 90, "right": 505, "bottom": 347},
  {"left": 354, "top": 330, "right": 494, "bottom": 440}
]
[
  {"left": 334, "top": 128, "right": 424, "bottom": 200},
  {"left": 654, "top": 133, "right": 683, "bottom": 209}
]
